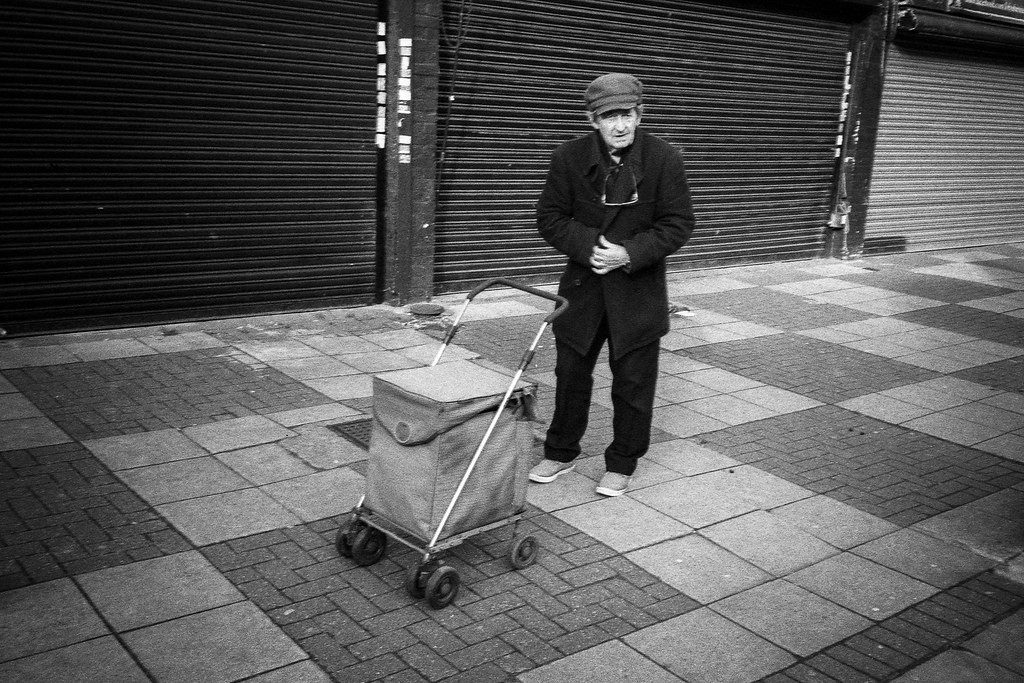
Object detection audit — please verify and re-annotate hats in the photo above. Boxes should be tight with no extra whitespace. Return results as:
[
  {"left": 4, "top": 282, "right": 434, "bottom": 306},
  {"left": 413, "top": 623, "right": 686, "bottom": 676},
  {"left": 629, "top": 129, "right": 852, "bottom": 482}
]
[{"left": 585, "top": 73, "right": 642, "bottom": 114}]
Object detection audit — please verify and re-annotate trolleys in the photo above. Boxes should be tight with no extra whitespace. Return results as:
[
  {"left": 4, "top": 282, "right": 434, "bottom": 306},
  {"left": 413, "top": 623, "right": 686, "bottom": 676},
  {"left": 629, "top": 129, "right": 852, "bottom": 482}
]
[{"left": 330, "top": 277, "right": 570, "bottom": 611}]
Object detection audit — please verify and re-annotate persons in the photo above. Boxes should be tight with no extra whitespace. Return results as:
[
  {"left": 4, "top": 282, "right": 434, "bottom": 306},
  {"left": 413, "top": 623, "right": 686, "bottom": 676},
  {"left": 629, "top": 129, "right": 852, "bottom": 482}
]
[{"left": 531, "top": 72, "right": 698, "bottom": 496}]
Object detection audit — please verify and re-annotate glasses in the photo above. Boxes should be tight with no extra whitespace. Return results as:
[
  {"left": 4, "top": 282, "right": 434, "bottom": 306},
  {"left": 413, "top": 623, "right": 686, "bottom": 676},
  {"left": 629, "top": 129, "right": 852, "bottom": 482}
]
[{"left": 601, "top": 165, "right": 638, "bottom": 206}]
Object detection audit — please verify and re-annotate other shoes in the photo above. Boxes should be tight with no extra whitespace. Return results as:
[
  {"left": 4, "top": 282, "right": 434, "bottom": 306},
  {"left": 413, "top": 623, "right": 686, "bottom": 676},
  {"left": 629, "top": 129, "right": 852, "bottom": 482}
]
[
  {"left": 595, "top": 472, "right": 633, "bottom": 497},
  {"left": 528, "top": 460, "right": 575, "bottom": 483}
]
